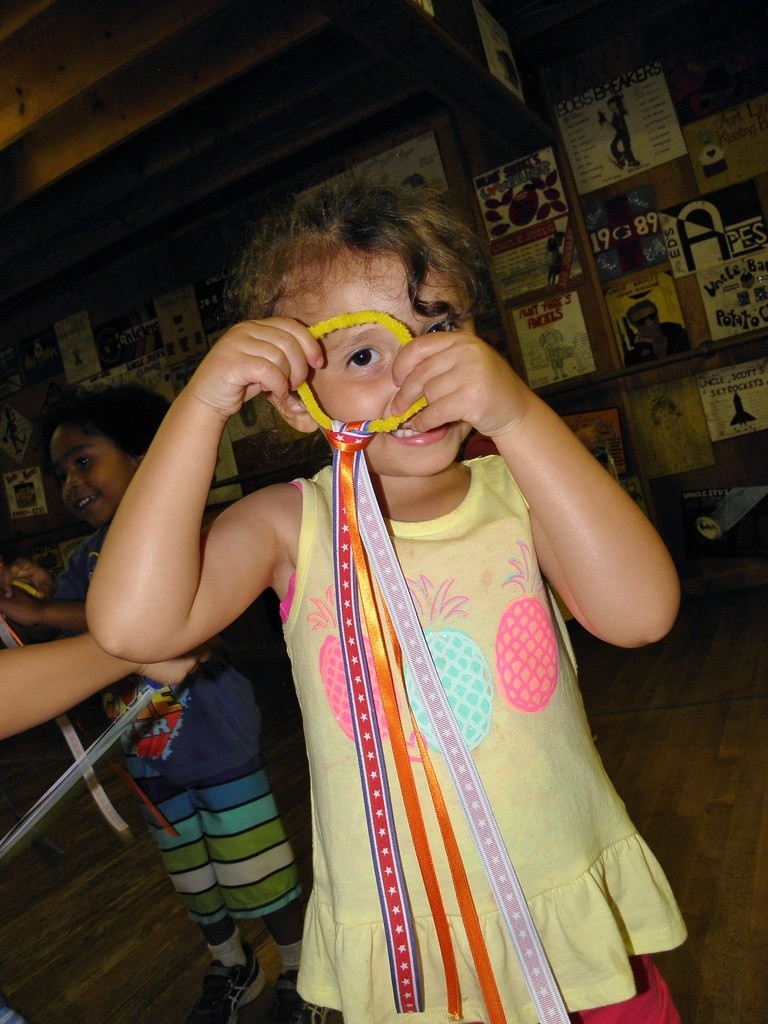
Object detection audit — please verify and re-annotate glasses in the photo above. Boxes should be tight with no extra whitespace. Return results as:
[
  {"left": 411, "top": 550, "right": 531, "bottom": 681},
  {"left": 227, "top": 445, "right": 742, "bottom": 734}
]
[{"left": 632, "top": 310, "right": 657, "bottom": 327}]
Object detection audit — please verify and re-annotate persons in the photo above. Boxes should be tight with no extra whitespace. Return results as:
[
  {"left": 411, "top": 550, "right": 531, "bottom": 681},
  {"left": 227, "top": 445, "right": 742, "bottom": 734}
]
[
  {"left": 0, "top": 606, "right": 203, "bottom": 1024},
  {"left": 0, "top": 95, "right": 768, "bottom": 518},
  {"left": 86, "top": 175, "right": 682, "bottom": 1024},
  {"left": 0, "top": 375, "right": 343, "bottom": 1024}
]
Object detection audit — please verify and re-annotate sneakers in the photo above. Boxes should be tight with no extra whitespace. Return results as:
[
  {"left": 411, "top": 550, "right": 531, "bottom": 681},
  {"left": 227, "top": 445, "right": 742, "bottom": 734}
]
[
  {"left": 186, "top": 941, "right": 265, "bottom": 1024},
  {"left": 269, "top": 968, "right": 330, "bottom": 1024}
]
